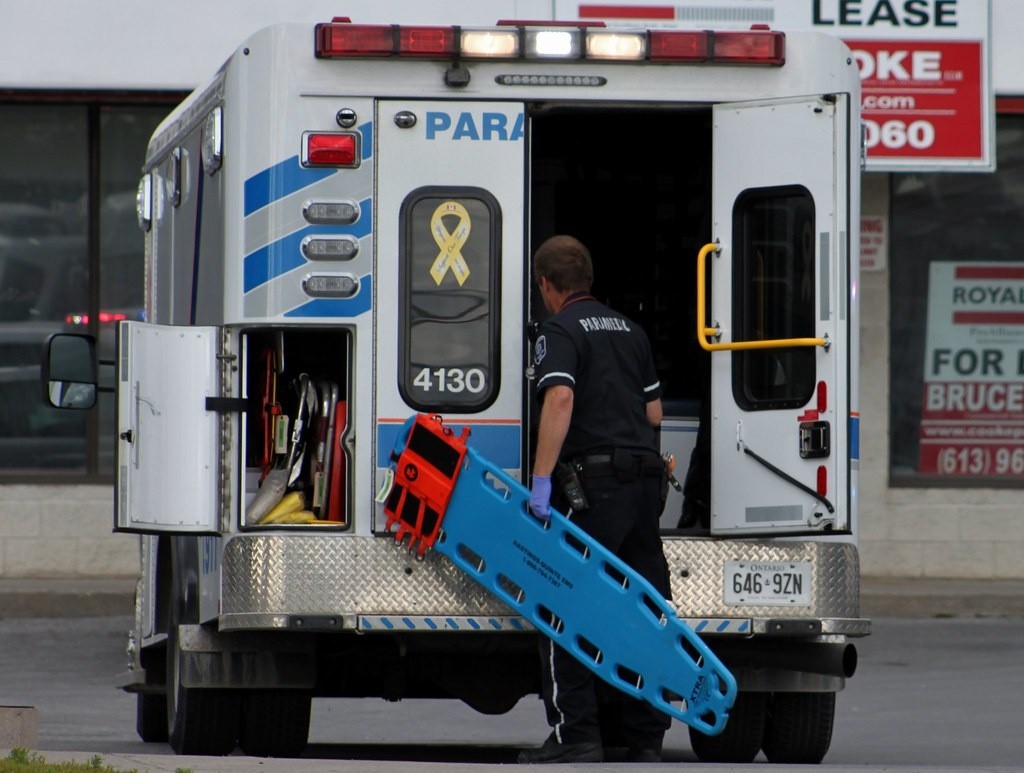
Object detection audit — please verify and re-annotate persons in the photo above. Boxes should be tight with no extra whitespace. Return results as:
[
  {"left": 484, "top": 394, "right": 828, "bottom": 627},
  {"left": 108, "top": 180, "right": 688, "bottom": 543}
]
[{"left": 517, "top": 236, "right": 682, "bottom": 764}]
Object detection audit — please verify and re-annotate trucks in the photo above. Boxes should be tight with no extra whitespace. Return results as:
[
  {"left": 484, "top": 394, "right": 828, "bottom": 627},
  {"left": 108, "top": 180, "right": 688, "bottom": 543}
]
[{"left": 38, "top": 17, "right": 871, "bottom": 763}]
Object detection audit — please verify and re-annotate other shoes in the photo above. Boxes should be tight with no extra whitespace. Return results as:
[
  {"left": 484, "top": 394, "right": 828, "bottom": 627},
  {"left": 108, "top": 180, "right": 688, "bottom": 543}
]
[
  {"left": 603, "top": 738, "right": 661, "bottom": 762},
  {"left": 517, "top": 728, "right": 602, "bottom": 762}
]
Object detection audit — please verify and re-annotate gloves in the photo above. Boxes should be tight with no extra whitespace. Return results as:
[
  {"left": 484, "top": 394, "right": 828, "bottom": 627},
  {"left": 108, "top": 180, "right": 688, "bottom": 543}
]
[{"left": 530, "top": 475, "right": 551, "bottom": 519}]
